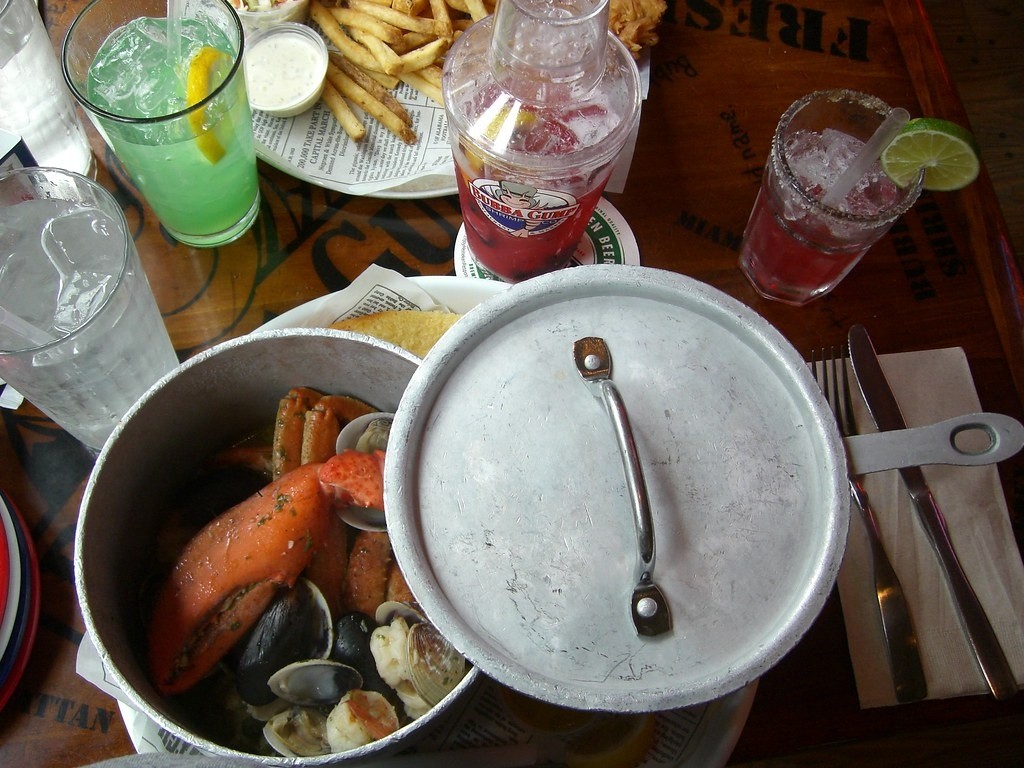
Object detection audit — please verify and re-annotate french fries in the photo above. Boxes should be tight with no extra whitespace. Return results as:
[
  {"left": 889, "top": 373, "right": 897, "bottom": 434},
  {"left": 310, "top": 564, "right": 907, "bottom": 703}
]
[{"left": 310, "top": 1, "right": 496, "bottom": 142}]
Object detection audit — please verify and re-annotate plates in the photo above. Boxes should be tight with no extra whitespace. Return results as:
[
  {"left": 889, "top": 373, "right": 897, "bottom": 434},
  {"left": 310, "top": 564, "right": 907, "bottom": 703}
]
[
  {"left": 119, "top": 276, "right": 758, "bottom": 768},
  {"left": 169, "top": 0, "right": 655, "bottom": 200}
]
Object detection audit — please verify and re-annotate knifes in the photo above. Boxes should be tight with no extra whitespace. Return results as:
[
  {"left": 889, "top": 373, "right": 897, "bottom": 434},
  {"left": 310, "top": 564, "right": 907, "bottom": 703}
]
[{"left": 847, "top": 321, "right": 1018, "bottom": 700}]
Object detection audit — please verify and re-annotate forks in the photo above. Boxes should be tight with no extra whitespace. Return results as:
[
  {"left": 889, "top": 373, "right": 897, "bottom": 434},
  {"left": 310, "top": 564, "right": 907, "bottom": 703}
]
[{"left": 802, "top": 346, "right": 928, "bottom": 706}]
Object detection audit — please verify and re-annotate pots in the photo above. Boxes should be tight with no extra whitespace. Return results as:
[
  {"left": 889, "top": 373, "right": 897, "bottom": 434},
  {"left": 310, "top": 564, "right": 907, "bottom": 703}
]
[{"left": 74, "top": 325, "right": 480, "bottom": 768}]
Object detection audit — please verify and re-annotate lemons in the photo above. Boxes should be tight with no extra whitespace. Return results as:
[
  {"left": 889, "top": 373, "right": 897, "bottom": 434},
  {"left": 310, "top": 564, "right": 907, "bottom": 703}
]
[{"left": 881, "top": 119, "right": 981, "bottom": 193}]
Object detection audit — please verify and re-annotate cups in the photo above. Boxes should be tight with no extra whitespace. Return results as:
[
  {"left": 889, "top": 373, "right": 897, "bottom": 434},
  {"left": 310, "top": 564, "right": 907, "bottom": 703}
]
[
  {"left": 0, "top": 0, "right": 100, "bottom": 207},
  {"left": 441, "top": 2, "right": 643, "bottom": 284},
  {"left": 59, "top": 1, "right": 263, "bottom": 248},
  {"left": 0, "top": 166, "right": 183, "bottom": 458},
  {"left": 738, "top": 88, "right": 929, "bottom": 307}
]
